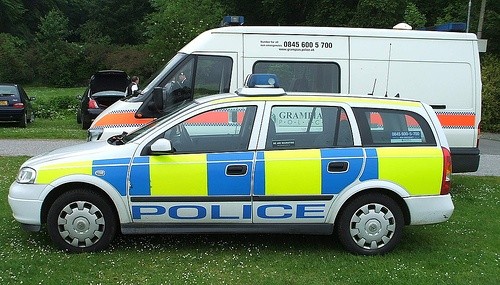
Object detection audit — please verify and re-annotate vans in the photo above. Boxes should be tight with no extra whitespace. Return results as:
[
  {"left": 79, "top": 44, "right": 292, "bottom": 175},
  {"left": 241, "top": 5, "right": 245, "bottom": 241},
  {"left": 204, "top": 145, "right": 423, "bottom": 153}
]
[{"left": 88, "top": 15, "right": 482, "bottom": 174}]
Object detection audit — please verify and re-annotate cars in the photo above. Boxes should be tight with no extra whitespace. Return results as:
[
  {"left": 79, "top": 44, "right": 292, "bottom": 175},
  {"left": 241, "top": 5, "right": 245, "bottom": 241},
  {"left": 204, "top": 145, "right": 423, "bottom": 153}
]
[
  {"left": 7, "top": 73, "right": 455, "bottom": 256},
  {"left": 0, "top": 84, "right": 37, "bottom": 127},
  {"left": 77, "top": 69, "right": 130, "bottom": 129}
]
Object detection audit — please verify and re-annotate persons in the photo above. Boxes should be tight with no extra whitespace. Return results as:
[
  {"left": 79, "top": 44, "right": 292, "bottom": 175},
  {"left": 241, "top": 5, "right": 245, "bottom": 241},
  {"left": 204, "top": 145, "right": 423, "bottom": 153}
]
[
  {"left": 178, "top": 73, "right": 189, "bottom": 87},
  {"left": 125, "top": 76, "right": 139, "bottom": 97}
]
